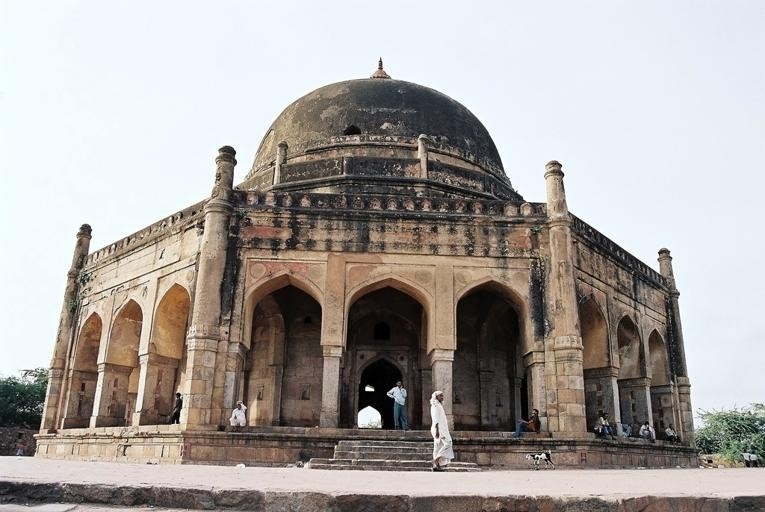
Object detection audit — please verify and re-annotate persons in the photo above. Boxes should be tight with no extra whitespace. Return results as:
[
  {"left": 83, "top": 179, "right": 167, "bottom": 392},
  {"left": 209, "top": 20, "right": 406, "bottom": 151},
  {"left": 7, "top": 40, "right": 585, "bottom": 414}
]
[
  {"left": 740, "top": 450, "right": 758, "bottom": 467},
  {"left": 621, "top": 421, "right": 633, "bottom": 437},
  {"left": 639, "top": 421, "right": 656, "bottom": 443},
  {"left": 513, "top": 409, "right": 541, "bottom": 438},
  {"left": 386, "top": 378, "right": 412, "bottom": 432},
  {"left": 665, "top": 423, "right": 682, "bottom": 446},
  {"left": 16, "top": 443, "right": 24, "bottom": 455},
  {"left": 170, "top": 392, "right": 182, "bottom": 424},
  {"left": 593, "top": 413, "right": 618, "bottom": 440},
  {"left": 429, "top": 390, "right": 455, "bottom": 471},
  {"left": 229, "top": 400, "right": 247, "bottom": 427}
]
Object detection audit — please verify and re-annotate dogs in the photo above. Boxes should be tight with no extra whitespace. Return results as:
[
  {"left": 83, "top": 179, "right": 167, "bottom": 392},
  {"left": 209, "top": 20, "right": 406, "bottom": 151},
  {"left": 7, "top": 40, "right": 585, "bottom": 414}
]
[{"left": 524, "top": 450, "right": 557, "bottom": 470}]
